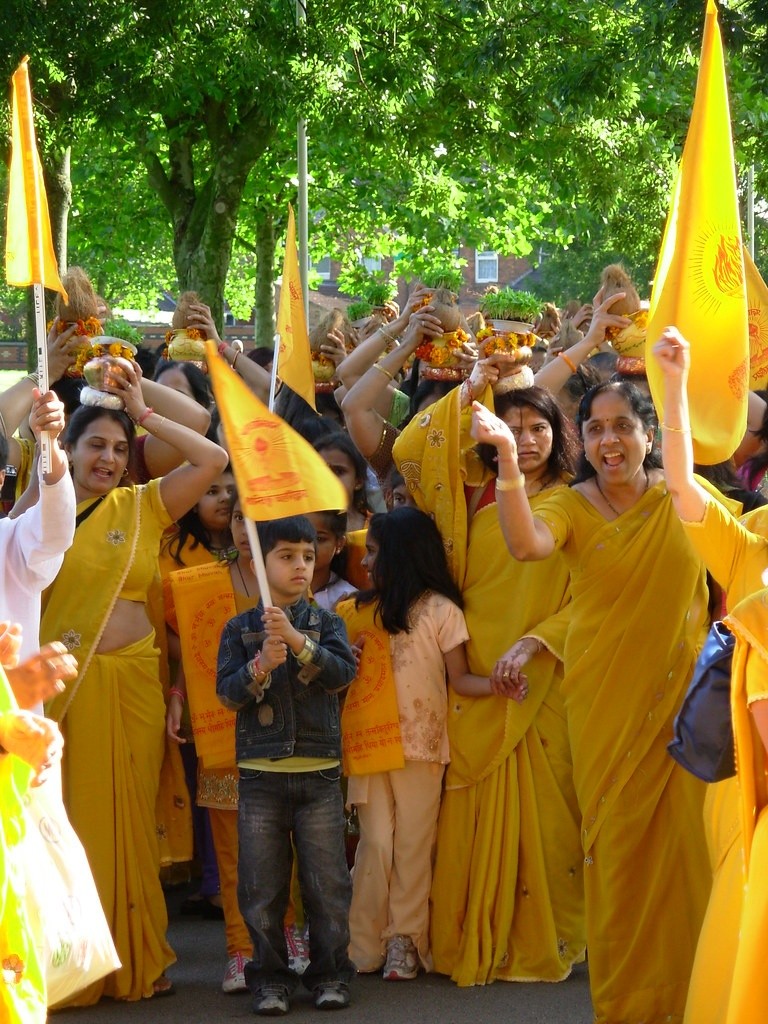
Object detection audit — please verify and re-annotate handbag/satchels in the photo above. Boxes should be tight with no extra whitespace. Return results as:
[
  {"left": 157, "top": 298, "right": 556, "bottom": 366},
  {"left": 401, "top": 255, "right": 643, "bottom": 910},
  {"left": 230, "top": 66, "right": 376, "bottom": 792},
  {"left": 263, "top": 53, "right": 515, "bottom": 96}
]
[{"left": 668, "top": 621, "right": 738, "bottom": 783}]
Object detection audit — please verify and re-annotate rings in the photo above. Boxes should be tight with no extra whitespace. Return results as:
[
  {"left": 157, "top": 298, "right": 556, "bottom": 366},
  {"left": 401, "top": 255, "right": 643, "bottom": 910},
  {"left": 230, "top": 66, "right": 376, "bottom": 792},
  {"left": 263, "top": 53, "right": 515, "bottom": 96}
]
[
  {"left": 502, "top": 670, "right": 510, "bottom": 678},
  {"left": 421, "top": 321, "right": 425, "bottom": 327},
  {"left": 124, "top": 383, "right": 132, "bottom": 391}
]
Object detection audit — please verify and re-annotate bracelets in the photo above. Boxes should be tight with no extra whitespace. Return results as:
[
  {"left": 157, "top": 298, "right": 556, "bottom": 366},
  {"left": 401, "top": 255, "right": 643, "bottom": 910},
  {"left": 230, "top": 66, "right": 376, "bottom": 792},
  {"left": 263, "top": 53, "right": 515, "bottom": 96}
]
[
  {"left": 230, "top": 347, "right": 241, "bottom": 373},
  {"left": 168, "top": 687, "right": 185, "bottom": 706},
  {"left": 252, "top": 650, "right": 271, "bottom": 677},
  {"left": 558, "top": 352, "right": 578, "bottom": 374},
  {"left": 376, "top": 331, "right": 392, "bottom": 348},
  {"left": 217, "top": 341, "right": 229, "bottom": 355},
  {"left": 494, "top": 472, "right": 526, "bottom": 492},
  {"left": 491, "top": 454, "right": 519, "bottom": 463},
  {"left": 22, "top": 371, "right": 39, "bottom": 386},
  {"left": 148, "top": 416, "right": 168, "bottom": 436},
  {"left": 466, "top": 378, "right": 475, "bottom": 406},
  {"left": 378, "top": 327, "right": 396, "bottom": 341},
  {"left": 131, "top": 407, "right": 154, "bottom": 428},
  {"left": 660, "top": 421, "right": 692, "bottom": 434},
  {"left": 372, "top": 363, "right": 394, "bottom": 381}
]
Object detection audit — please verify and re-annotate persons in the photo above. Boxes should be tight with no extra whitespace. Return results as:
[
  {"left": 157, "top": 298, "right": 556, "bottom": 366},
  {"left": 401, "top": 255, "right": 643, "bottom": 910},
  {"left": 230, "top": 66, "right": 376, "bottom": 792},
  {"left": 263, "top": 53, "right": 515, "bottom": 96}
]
[{"left": 0, "top": 283, "right": 768, "bottom": 1024}]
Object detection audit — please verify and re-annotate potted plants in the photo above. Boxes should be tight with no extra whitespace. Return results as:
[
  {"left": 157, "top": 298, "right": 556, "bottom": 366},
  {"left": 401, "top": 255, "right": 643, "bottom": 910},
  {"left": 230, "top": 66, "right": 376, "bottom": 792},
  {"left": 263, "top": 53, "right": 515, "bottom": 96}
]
[
  {"left": 477, "top": 284, "right": 546, "bottom": 336},
  {"left": 365, "top": 279, "right": 398, "bottom": 315},
  {"left": 169, "top": 291, "right": 208, "bottom": 361},
  {"left": 83, "top": 313, "right": 142, "bottom": 389},
  {"left": 346, "top": 302, "right": 375, "bottom": 331},
  {"left": 416, "top": 265, "right": 467, "bottom": 305}
]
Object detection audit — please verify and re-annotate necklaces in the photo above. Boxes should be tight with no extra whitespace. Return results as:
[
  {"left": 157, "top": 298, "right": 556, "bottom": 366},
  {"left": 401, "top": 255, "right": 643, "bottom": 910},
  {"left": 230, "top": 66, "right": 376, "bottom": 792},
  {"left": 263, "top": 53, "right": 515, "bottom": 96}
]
[
  {"left": 595, "top": 468, "right": 649, "bottom": 517},
  {"left": 205, "top": 546, "right": 239, "bottom": 558},
  {"left": 235, "top": 557, "right": 251, "bottom": 598}
]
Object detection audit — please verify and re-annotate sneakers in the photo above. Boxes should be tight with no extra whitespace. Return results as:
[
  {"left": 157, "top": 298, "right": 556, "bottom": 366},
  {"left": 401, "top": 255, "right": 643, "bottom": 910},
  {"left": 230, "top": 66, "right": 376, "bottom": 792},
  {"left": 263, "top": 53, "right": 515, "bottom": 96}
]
[
  {"left": 253, "top": 983, "right": 289, "bottom": 1014},
  {"left": 383, "top": 935, "right": 420, "bottom": 980},
  {"left": 284, "top": 924, "right": 310, "bottom": 975},
  {"left": 315, "top": 979, "right": 351, "bottom": 1007},
  {"left": 221, "top": 956, "right": 253, "bottom": 991}
]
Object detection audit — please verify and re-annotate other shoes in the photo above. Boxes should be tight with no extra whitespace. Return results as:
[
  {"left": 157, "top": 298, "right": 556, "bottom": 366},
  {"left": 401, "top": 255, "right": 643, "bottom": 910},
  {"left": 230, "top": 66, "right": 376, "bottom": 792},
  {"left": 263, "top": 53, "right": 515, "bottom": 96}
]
[{"left": 152, "top": 978, "right": 174, "bottom": 996}]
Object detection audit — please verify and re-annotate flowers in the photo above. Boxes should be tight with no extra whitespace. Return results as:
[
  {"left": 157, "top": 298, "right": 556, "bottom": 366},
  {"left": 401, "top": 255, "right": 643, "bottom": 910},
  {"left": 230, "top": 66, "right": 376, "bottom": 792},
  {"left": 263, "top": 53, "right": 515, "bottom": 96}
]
[
  {"left": 45, "top": 317, "right": 103, "bottom": 337},
  {"left": 162, "top": 348, "right": 168, "bottom": 359},
  {"left": 186, "top": 327, "right": 202, "bottom": 341},
  {"left": 605, "top": 314, "right": 629, "bottom": 341},
  {"left": 476, "top": 326, "right": 537, "bottom": 355},
  {"left": 164, "top": 330, "right": 176, "bottom": 345},
  {"left": 412, "top": 295, "right": 432, "bottom": 313},
  {"left": 75, "top": 342, "right": 134, "bottom": 371},
  {"left": 415, "top": 327, "right": 471, "bottom": 365}
]
